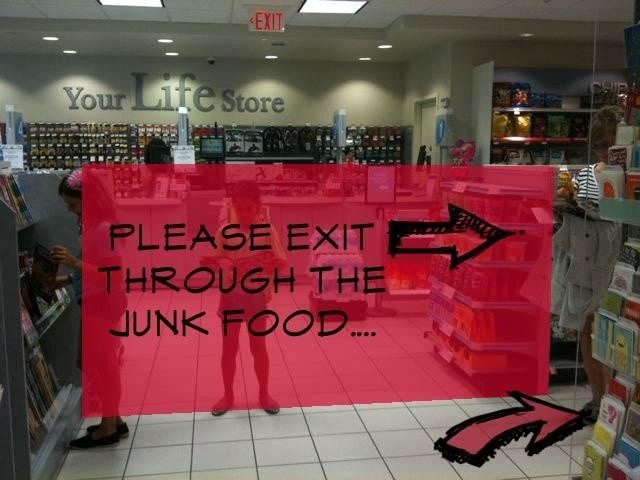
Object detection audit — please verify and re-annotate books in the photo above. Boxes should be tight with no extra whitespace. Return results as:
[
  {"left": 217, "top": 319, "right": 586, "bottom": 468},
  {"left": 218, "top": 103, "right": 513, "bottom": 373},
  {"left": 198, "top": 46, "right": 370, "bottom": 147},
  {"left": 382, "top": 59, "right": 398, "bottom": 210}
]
[
  {"left": 580, "top": 1, "right": 640, "bottom": 480},
  {"left": 1, "top": 173, "right": 71, "bottom": 459}
]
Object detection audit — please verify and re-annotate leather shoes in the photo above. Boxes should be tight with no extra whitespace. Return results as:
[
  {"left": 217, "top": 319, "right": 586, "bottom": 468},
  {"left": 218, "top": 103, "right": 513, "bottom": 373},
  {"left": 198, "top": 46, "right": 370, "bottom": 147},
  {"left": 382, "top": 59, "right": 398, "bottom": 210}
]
[{"left": 258, "top": 395, "right": 280, "bottom": 414}]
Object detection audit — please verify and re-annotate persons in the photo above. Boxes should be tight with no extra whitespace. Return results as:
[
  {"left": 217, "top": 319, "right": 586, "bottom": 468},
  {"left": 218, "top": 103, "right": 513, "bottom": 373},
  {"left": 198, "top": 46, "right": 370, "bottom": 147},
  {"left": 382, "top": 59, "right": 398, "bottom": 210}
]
[
  {"left": 202, "top": 178, "right": 288, "bottom": 416},
  {"left": 124, "top": 138, "right": 171, "bottom": 197},
  {"left": 556, "top": 106, "right": 625, "bottom": 422},
  {"left": 48, "top": 167, "right": 129, "bottom": 451}
]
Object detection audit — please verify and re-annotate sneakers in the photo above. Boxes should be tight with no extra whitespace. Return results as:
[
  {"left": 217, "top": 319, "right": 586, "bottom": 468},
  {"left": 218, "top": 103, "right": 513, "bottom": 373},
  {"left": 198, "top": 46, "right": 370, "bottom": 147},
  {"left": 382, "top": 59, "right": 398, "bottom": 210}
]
[
  {"left": 69, "top": 433, "right": 119, "bottom": 450},
  {"left": 211, "top": 397, "right": 234, "bottom": 416},
  {"left": 86, "top": 422, "right": 130, "bottom": 439},
  {"left": 581, "top": 400, "right": 600, "bottom": 423}
]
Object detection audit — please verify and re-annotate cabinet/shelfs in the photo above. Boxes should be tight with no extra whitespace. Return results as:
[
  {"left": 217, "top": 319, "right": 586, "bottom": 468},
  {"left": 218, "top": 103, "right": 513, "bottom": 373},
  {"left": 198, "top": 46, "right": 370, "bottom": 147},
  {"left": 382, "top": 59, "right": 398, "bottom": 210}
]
[{"left": 0, "top": 60, "right": 640, "bottom": 480}]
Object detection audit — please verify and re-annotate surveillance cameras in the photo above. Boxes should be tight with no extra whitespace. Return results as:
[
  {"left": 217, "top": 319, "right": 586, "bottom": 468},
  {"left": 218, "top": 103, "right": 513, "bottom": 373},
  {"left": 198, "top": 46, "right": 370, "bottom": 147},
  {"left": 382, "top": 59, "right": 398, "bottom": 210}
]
[{"left": 207, "top": 57, "right": 216, "bottom": 64}]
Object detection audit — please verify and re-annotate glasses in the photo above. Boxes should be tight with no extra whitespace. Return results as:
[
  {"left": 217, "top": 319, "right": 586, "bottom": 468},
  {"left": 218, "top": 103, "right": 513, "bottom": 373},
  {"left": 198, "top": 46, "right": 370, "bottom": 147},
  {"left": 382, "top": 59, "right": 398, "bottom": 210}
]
[{"left": 587, "top": 141, "right": 609, "bottom": 150}]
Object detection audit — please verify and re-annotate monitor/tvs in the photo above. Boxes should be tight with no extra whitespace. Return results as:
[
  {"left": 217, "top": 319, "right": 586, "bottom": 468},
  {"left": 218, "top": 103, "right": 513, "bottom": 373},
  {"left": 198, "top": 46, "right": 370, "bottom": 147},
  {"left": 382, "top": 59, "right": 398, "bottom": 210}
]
[
  {"left": 199, "top": 138, "right": 226, "bottom": 161},
  {"left": 415, "top": 145, "right": 427, "bottom": 172}
]
